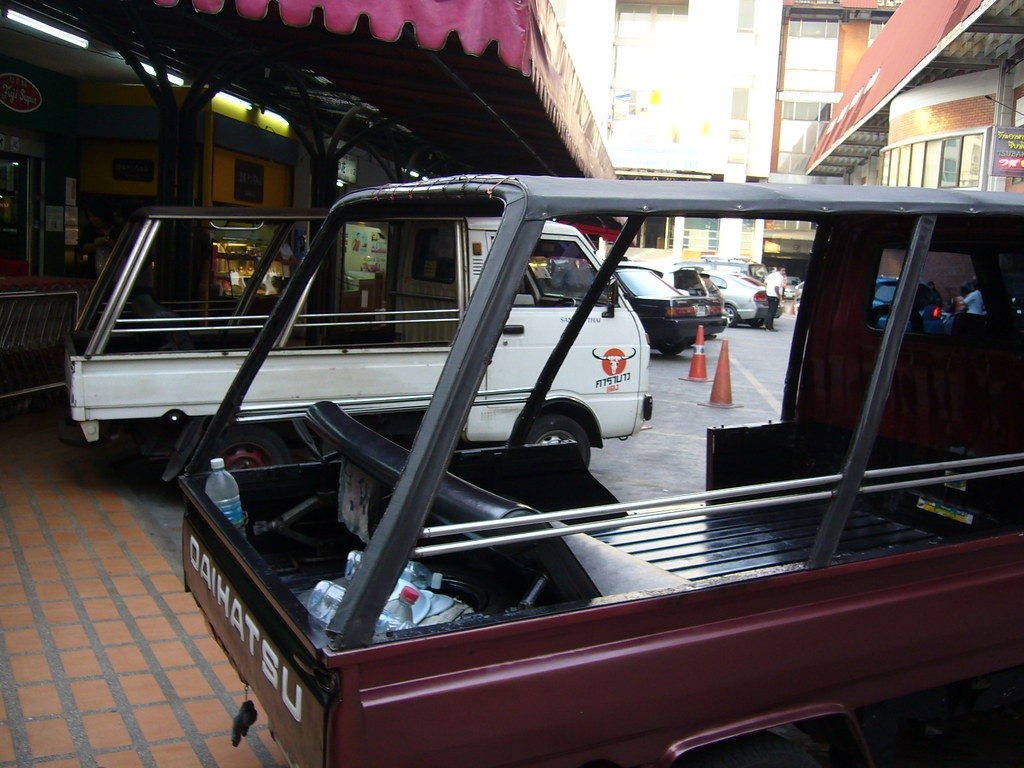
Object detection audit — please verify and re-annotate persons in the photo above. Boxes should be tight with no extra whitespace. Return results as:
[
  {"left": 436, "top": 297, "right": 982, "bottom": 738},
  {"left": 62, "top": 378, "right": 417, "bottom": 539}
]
[
  {"left": 272, "top": 230, "right": 301, "bottom": 294},
  {"left": 960, "top": 289, "right": 983, "bottom": 315},
  {"left": 764, "top": 267, "right": 786, "bottom": 332}
]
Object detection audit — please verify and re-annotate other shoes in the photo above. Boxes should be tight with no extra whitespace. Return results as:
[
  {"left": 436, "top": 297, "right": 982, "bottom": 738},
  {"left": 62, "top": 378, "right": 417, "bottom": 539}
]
[{"left": 766, "top": 328, "right": 778, "bottom": 332}]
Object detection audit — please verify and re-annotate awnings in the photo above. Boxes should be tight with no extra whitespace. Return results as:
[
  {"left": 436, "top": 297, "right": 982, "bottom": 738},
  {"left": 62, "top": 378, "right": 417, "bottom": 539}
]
[{"left": 80, "top": 1, "right": 617, "bottom": 210}]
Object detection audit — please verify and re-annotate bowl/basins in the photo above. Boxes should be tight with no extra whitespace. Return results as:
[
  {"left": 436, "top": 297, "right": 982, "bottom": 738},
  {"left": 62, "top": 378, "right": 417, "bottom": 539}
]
[{"left": 387, "top": 578, "right": 430, "bottom": 625}]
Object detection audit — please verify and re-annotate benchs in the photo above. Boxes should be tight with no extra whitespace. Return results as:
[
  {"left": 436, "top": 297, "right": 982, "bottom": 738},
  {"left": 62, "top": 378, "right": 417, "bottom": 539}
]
[
  {"left": 136, "top": 295, "right": 194, "bottom": 352},
  {"left": 307, "top": 394, "right": 692, "bottom": 599}
]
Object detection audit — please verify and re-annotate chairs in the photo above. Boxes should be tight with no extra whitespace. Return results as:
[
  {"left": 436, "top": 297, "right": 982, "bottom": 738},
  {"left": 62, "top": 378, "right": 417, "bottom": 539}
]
[{"left": 950, "top": 313, "right": 989, "bottom": 337}]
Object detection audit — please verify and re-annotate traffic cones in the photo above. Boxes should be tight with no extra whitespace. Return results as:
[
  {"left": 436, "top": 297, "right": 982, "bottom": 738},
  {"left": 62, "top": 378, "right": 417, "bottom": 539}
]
[
  {"left": 679, "top": 325, "right": 715, "bottom": 383},
  {"left": 787, "top": 302, "right": 797, "bottom": 316},
  {"left": 697, "top": 339, "right": 745, "bottom": 409}
]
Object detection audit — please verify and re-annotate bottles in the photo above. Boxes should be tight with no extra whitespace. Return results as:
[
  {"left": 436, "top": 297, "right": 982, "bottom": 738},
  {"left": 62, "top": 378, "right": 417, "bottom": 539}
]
[
  {"left": 382, "top": 585, "right": 419, "bottom": 622},
  {"left": 518, "top": 574, "right": 548, "bottom": 610},
  {"left": 307, "top": 580, "right": 418, "bottom": 639},
  {"left": 345, "top": 550, "right": 443, "bottom": 589},
  {"left": 205, "top": 458, "right": 246, "bottom": 537}
]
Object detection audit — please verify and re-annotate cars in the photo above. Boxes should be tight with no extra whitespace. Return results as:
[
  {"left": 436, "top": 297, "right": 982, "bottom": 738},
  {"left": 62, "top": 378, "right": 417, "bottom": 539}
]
[
  {"left": 702, "top": 271, "right": 785, "bottom": 328},
  {"left": 555, "top": 267, "right": 730, "bottom": 356},
  {"left": 619, "top": 261, "right": 729, "bottom": 333},
  {"left": 783, "top": 277, "right": 801, "bottom": 299},
  {"left": 870, "top": 274, "right": 947, "bottom": 335}
]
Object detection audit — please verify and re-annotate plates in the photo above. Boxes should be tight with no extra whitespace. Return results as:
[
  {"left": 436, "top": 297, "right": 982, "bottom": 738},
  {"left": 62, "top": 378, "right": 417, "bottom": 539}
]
[{"left": 419, "top": 590, "right": 454, "bottom": 617}]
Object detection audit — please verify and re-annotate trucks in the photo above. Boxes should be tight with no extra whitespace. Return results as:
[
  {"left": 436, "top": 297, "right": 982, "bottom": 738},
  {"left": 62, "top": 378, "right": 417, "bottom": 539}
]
[
  {"left": 176, "top": 174, "right": 1023, "bottom": 768},
  {"left": 62, "top": 205, "right": 656, "bottom": 471}
]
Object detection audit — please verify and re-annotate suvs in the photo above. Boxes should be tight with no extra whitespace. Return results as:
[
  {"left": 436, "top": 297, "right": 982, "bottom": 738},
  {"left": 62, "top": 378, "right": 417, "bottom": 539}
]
[{"left": 673, "top": 256, "right": 769, "bottom": 284}]
